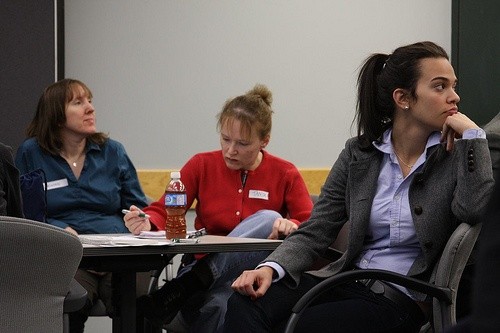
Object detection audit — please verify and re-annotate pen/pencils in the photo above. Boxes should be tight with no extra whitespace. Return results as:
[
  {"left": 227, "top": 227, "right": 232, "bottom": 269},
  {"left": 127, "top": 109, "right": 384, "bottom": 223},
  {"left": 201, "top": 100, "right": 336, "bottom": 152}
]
[
  {"left": 121, "top": 209, "right": 151, "bottom": 218},
  {"left": 170, "top": 238, "right": 200, "bottom": 243}
]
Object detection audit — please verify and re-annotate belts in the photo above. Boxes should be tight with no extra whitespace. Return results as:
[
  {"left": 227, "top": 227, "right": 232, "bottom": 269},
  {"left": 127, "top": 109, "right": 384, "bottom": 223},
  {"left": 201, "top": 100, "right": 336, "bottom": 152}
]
[{"left": 348, "top": 266, "right": 418, "bottom": 314}]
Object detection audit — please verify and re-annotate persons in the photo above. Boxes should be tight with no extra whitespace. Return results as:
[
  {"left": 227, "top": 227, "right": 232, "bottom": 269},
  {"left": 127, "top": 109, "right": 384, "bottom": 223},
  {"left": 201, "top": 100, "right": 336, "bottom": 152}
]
[
  {"left": 14, "top": 77, "right": 148, "bottom": 333},
  {"left": 123, "top": 84, "right": 315, "bottom": 332},
  {"left": 222, "top": 40, "right": 494, "bottom": 332}
]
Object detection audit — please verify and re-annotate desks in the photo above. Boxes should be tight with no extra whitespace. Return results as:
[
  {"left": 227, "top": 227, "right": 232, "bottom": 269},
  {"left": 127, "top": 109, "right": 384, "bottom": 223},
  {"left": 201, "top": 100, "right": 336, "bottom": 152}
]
[{"left": 79, "top": 234, "right": 284, "bottom": 333}]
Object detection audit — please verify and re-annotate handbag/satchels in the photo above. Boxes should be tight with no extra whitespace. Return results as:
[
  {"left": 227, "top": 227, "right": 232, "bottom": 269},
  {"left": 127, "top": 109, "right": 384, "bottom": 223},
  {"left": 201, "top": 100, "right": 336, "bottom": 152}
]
[{"left": 21, "top": 168, "right": 48, "bottom": 223}]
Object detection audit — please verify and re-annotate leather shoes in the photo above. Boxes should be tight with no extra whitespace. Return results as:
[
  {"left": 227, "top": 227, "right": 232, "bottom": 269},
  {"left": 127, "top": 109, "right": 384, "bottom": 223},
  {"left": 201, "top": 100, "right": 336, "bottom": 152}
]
[{"left": 136, "top": 277, "right": 191, "bottom": 326}]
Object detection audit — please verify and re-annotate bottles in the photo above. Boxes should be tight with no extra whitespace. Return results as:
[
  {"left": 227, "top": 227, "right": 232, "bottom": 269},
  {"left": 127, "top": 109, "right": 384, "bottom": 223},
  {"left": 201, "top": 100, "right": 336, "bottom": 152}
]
[{"left": 164, "top": 171, "right": 187, "bottom": 239}]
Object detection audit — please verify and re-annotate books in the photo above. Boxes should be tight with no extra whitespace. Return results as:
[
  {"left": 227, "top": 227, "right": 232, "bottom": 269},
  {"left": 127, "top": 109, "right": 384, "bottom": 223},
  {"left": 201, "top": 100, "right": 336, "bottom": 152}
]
[{"left": 135, "top": 226, "right": 206, "bottom": 239}]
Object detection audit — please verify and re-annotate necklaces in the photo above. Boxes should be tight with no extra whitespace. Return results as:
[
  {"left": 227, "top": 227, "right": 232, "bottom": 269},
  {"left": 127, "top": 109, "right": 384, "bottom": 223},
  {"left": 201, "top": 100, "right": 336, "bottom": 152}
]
[
  {"left": 56, "top": 143, "right": 84, "bottom": 167},
  {"left": 390, "top": 139, "right": 422, "bottom": 168}
]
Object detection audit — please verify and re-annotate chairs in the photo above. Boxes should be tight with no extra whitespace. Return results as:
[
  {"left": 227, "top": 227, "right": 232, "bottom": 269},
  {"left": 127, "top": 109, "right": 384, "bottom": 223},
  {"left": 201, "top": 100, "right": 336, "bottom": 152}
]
[
  {"left": 284, "top": 220, "right": 483, "bottom": 333},
  {"left": 0, "top": 216, "right": 87, "bottom": 333}
]
